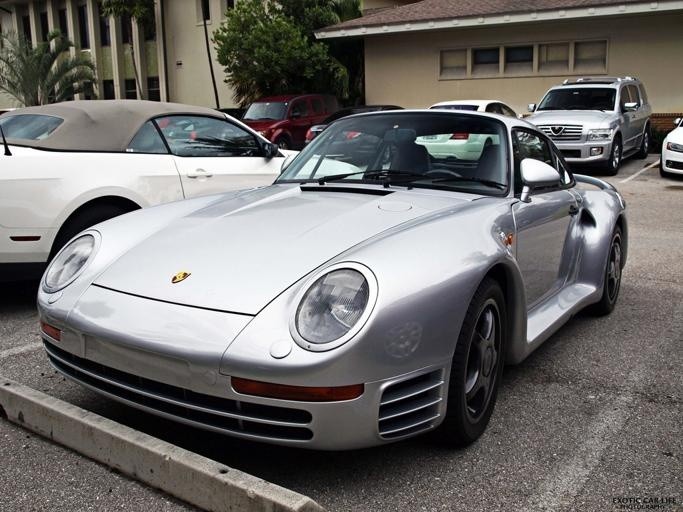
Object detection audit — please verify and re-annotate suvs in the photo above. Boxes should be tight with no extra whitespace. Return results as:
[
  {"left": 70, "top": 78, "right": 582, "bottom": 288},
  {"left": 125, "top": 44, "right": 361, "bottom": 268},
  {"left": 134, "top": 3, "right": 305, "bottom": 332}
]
[
  {"left": 520, "top": 76, "right": 652, "bottom": 176},
  {"left": 242, "top": 92, "right": 337, "bottom": 153}
]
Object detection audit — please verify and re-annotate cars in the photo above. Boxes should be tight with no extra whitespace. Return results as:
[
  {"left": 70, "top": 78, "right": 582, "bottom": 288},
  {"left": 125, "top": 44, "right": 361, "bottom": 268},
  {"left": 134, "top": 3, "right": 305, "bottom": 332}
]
[
  {"left": 659, "top": 116, "right": 683, "bottom": 178},
  {"left": 413, "top": 100, "right": 522, "bottom": 162},
  {"left": 302, "top": 104, "right": 405, "bottom": 152}
]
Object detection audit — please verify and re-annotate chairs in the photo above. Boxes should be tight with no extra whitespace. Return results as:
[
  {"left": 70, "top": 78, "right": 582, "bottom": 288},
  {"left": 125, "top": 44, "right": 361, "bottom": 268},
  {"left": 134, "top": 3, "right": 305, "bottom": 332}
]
[{"left": 383, "top": 139, "right": 433, "bottom": 184}]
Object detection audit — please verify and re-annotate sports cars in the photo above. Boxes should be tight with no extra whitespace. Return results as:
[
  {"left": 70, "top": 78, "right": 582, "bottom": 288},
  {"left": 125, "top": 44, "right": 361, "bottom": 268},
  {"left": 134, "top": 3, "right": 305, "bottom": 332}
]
[
  {"left": 36, "top": 107, "right": 628, "bottom": 449},
  {"left": 0, "top": 97, "right": 365, "bottom": 286}
]
